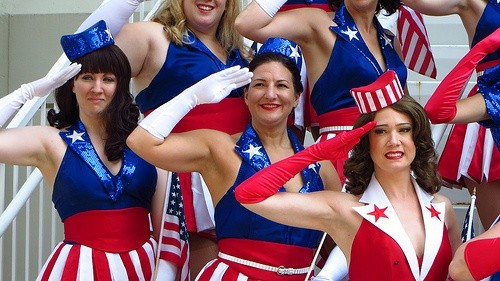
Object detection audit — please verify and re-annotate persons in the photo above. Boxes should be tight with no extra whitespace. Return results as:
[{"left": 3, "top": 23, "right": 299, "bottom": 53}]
[
  {"left": 0, "top": 18, "right": 169, "bottom": 281},
  {"left": 74, "top": 0, "right": 500, "bottom": 281}
]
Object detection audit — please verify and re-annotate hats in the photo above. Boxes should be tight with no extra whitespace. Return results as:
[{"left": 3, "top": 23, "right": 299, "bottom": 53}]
[
  {"left": 350, "top": 69, "right": 404, "bottom": 114},
  {"left": 61, "top": 20, "right": 115, "bottom": 62},
  {"left": 258, "top": 37, "right": 302, "bottom": 72}
]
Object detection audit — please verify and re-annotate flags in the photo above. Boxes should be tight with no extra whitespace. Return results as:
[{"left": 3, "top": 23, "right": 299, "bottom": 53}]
[
  {"left": 396, "top": 2, "right": 438, "bottom": 79},
  {"left": 459, "top": 202, "right": 476, "bottom": 245},
  {"left": 159, "top": 171, "right": 191, "bottom": 281}
]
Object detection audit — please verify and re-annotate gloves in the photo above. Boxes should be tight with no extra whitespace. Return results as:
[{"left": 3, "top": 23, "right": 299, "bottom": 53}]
[
  {"left": 424, "top": 28, "right": 500, "bottom": 124},
  {"left": 235, "top": 120, "right": 378, "bottom": 203},
  {"left": 0, "top": 63, "right": 82, "bottom": 127},
  {"left": 139, "top": 64, "right": 253, "bottom": 140}
]
